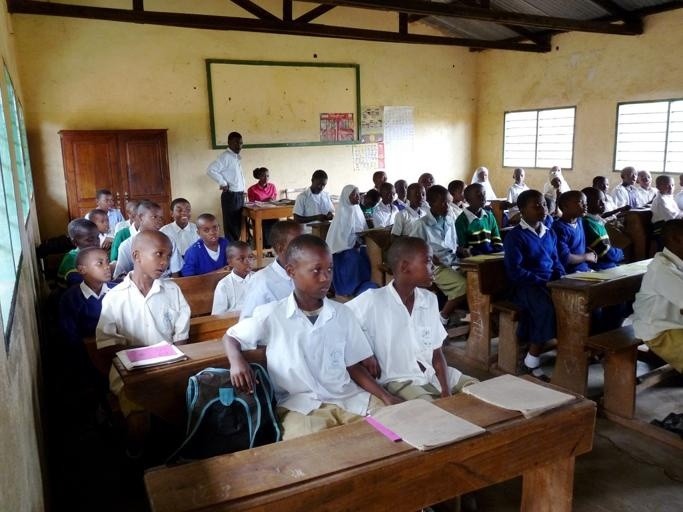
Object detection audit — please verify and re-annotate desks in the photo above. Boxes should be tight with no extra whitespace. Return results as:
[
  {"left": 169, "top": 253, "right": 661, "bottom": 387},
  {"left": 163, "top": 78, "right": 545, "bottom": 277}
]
[{"left": 71, "top": 188, "right": 682, "bottom": 510}]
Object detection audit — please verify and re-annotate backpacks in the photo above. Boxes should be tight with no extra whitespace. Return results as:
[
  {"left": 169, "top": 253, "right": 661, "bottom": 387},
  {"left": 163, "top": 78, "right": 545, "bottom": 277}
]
[{"left": 165, "top": 361, "right": 281, "bottom": 465}]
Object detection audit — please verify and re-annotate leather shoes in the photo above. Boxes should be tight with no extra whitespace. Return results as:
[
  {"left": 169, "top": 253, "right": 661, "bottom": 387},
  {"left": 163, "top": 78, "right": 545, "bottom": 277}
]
[{"left": 519, "top": 357, "right": 550, "bottom": 383}]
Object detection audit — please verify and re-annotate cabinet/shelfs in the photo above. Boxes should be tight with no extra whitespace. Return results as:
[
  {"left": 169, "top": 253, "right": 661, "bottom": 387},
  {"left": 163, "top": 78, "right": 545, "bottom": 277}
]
[{"left": 58, "top": 124, "right": 174, "bottom": 233}]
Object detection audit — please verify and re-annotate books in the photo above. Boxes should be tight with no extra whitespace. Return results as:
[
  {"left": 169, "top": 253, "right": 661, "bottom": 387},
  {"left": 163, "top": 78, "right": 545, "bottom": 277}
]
[
  {"left": 112, "top": 339, "right": 188, "bottom": 372},
  {"left": 459, "top": 372, "right": 578, "bottom": 420},
  {"left": 366, "top": 395, "right": 487, "bottom": 452}
]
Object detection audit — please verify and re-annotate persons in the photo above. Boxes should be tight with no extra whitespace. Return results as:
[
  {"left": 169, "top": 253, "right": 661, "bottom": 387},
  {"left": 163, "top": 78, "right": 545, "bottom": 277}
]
[
  {"left": 56, "top": 189, "right": 258, "bottom": 317},
  {"left": 206, "top": 131, "right": 248, "bottom": 243},
  {"left": 51, "top": 245, "right": 114, "bottom": 353},
  {"left": 93, "top": 229, "right": 192, "bottom": 479},
  {"left": 221, "top": 234, "right": 405, "bottom": 439},
  {"left": 239, "top": 164, "right": 683, "bottom": 436},
  {"left": 341, "top": 235, "right": 481, "bottom": 512}
]
